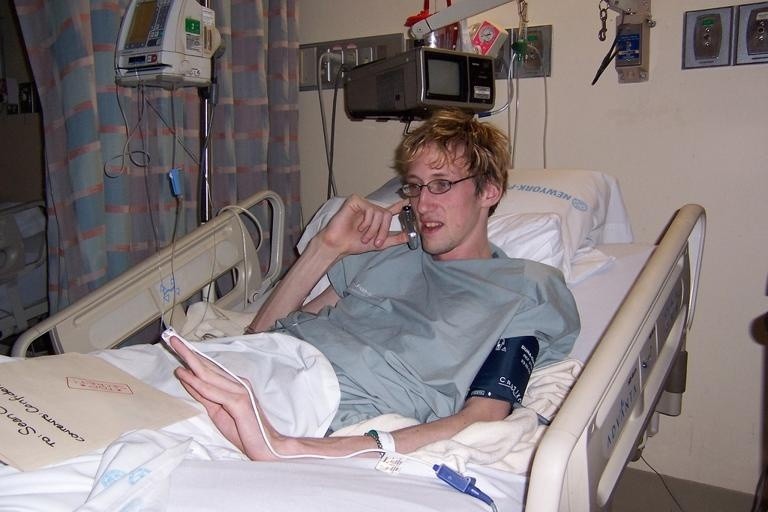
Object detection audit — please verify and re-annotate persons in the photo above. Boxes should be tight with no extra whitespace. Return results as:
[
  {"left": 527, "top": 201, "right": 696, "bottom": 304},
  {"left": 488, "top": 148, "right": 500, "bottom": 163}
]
[{"left": 168, "top": 108, "right": 581, "bottom": 470}]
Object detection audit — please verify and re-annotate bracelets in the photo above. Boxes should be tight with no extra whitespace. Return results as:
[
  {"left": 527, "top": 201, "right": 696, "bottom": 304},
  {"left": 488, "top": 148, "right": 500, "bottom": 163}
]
[{"left": 364, "top": 433, "right": 384, "bottom": 459}]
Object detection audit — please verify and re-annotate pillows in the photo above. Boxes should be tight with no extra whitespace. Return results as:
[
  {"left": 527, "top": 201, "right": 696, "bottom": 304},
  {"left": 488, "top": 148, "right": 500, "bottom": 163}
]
[
  {"left": 298, "top": 197, "right": 563, "bottom": 274},
  {"left": 363, "top": 170, "right": 633, "bottom": 256}
]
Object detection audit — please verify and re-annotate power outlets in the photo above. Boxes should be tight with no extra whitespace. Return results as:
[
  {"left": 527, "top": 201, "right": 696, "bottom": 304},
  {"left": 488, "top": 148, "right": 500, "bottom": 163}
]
[
  {"left": 7, "top": 81, "right": 39, "bottom": 114},
  {"left": 514, "top": 24, "right": 551, "bottom": 78},
  {"left": 681, "top": 7, "right": 734, "bottom": 71},
  {"left": 734, "top": 1, "right": 767, "bottom": 66},
  {"left": 315, "top": 33, "right": 374, "bottom": 91}
]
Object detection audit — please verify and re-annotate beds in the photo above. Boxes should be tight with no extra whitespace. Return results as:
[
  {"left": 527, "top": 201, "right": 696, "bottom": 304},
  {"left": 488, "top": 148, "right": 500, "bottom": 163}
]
[
  {"left": 0, "top": 195, "right": 49, "bottom": 344},
  {"left": 0, "top": 192, "right": 706, "bottom": 511}
]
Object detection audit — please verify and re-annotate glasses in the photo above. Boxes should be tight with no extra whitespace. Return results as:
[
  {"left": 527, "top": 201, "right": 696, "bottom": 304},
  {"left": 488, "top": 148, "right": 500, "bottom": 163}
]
[{"left": 400, "top": 174, "right": 478, "bottom": 197}]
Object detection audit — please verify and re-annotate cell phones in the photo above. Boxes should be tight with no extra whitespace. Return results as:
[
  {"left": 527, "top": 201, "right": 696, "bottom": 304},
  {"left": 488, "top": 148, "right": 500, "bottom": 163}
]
[{"left": 398, "top": 205, "right": 418, "bottom": 250}]
